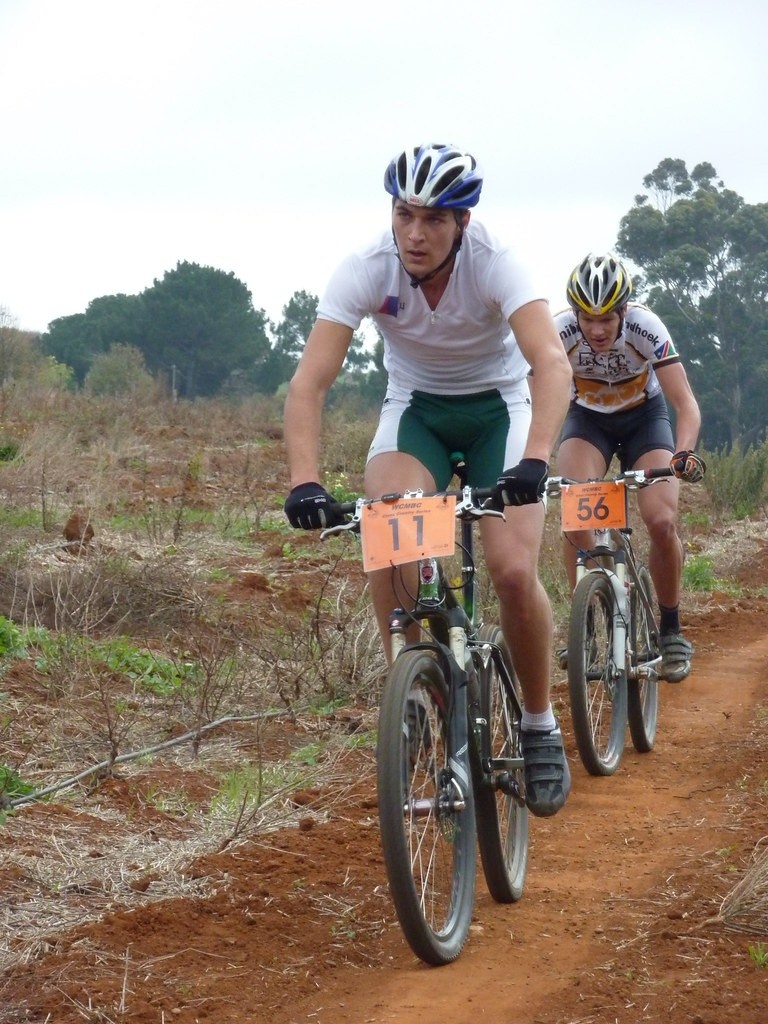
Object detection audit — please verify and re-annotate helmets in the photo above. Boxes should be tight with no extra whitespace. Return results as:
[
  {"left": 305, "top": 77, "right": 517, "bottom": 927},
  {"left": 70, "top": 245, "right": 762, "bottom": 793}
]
[
  {"left": 384, "top": 143, "right": 482, "bottom": 209},
  {"left": 567, "top": 252, "right": 632, "bottom": 316}
]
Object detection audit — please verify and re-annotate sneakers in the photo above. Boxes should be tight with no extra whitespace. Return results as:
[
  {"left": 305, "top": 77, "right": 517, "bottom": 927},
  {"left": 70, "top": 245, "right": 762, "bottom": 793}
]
[
  {"left": 520, "top": 717, "right": 571, "bottom": 818},
  {"left": 556, "top": 634, "right": 600, "bottom": 670},
  {"left": 403, "top": 698, "right": 431, "bottom": 769},
  {"left": 658, "top": 634, "right": 692, "bottom": 683}
]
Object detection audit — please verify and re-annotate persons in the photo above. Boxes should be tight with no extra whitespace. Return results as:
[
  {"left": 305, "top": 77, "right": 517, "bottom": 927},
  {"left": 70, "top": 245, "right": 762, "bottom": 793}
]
[
  {"left": 526, "top": 253, "right": 702, "bottom": 684},
  {"left": 286, "top": 141, "right": 571, "bottom": 818}
]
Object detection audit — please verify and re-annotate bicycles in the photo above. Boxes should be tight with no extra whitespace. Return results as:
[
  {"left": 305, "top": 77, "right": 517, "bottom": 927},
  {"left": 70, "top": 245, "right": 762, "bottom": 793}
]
[
  {"left": 556, "top": 461, "right": 669, "bottom": 779},
  {"left": 316, "top": 447, "right": 585, "bottom": 969}
]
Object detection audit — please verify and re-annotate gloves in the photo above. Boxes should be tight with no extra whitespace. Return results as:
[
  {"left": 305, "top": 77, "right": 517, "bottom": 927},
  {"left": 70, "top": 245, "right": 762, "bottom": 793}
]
[
  {"left": 496, "top": 458, "right": 549, "bottom": 506},
  {"left": 285, "top": 482, "right": 343, "bottom": 530},
  {"left": 670, "top": 450, "right": 705, "bottom": 482}
]
